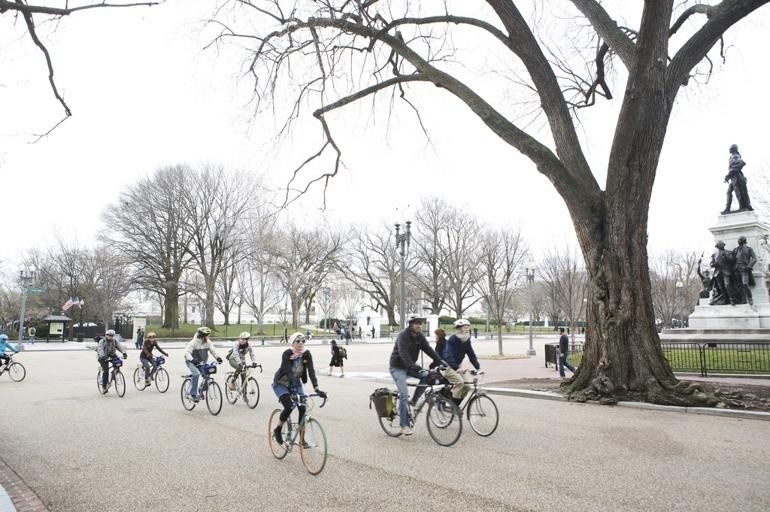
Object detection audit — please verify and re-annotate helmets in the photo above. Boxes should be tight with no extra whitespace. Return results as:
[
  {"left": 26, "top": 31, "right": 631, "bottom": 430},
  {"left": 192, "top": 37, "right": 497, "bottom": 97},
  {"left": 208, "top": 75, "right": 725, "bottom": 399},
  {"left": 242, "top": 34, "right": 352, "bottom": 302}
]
[
  {"left": 106, "top": 330, "right": 116, "bottom": 335},
  {"left": 199, "top": 327, "right": 211, "bottom": 334},
  {"left": 408, "top": 314, "right": 425, "bottom": 321},
  {"left": 2, "top": 334, "right": 8, "bottom": 339},
  {"left": 453, "top": 319, "right": 470, "bottom": 328},
  {"left": 147, "top": 332, "right": 156, "bottom": 338},
  {"left": 239, "top": 331, "right": 250, "bottom": 339}
]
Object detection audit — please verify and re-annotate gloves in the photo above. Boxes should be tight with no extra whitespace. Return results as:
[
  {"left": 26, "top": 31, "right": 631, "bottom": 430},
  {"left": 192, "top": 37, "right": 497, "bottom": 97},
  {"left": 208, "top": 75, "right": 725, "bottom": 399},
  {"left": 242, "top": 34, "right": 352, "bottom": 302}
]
[
  {"left": 439, "top": 359, "right": 448, "bottom": 370},
  {"left": 217, "top": 358, "right": 222, "bottom": 363},
  {"left": 418, "top": 369, "right": 429, "bottom": 376},
  {"left": 315, "top": 390, "right": 327, "bottom": 398},
  {"left": 290, "top": 388, "right": 298, "bottom": 395},
  {"left": 192, "top": 361, "right": 199, "bottom": 365}
]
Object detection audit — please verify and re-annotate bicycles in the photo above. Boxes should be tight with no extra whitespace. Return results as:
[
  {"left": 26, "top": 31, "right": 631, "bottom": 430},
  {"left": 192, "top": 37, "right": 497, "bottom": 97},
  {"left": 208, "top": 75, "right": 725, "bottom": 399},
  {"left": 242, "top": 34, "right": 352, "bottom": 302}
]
[
  {"left": 95, "top": 354, "right": 171, "bottom": 398},
  {"left": 180, "top": 359, "right": 263, "bottom": 416},
  {"left": 0, "top": 349, "right": 26, "bottom": 383},
  {"left": 374, "top": 364, "right": 500, "bottom": 447},
  {"left": 267, "top": 393, "right": 329, "bottom": 476}
]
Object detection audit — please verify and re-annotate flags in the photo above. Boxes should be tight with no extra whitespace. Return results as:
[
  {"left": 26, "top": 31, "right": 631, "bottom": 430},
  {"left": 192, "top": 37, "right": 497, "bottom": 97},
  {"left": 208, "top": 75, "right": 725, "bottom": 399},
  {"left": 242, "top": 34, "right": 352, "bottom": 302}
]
[{"left": 61, "top": 296, "right": 79, "bottom": 311}]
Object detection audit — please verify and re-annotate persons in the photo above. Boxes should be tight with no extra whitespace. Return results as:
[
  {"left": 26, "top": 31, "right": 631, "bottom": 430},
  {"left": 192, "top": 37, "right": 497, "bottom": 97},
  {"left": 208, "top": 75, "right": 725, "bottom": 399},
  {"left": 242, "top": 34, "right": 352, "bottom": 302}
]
[
  {"left": 227, "top": 333, "right": 255, "bottom": 401},
  {"left": 272, "top": 331, "right": 327, "bottom": 448},
  {"left": 28, "top": 326, "right": 36, "bottom": 344},
  {"left": 96, "top": 327, "right": 169, "bottom": 395},
  {"left": 721, "top": 144, "right": 752, "bottom": 213},
  {"left": 697, "top": 258, "right": 712, "bottom": 305},
  {"left": 710, "top": 241, "right": 736, "bottom": 305},
  {"left": 183, "top": 328, "right": 222, "bottom": 402},
  {"left": 733, "top": 237, "right": 757, "bottom": 305},
  {"left": 390, "top": 314, "right": 484, "bottom": 435},
  {"left": 557, "top": 327, "right": 576, "bottom": 378},
  {"left": 328, "top": 340, "right": 345, "bottom": 377},
  {"left": 0, "top": 334, "right": 19, "bottom": 370},
  {"left": 334, "top": 320, "right": 375, "bottom": 345}
]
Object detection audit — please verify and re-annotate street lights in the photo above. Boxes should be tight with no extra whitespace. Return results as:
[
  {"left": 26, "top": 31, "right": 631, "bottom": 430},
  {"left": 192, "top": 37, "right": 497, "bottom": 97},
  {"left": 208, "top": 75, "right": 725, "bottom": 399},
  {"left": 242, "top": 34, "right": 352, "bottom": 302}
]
[
  {"left": 525, "top": 268, "right": 536, "bottom": 355},
  {"left": 676, "top": 279, "right": 686, "bottom": 328},
  {"left": 14, "top": 270, "right": 34, "bottom": 352},
  {"left": 394, "top": 220, "right": 411, "bottom": 331}
]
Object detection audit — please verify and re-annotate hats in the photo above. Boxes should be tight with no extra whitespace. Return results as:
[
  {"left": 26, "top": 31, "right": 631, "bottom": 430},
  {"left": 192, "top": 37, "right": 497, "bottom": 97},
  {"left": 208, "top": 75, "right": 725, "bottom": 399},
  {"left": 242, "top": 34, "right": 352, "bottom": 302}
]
[{"left": 288, "top": 332, "right": 304, "bottom": 344}]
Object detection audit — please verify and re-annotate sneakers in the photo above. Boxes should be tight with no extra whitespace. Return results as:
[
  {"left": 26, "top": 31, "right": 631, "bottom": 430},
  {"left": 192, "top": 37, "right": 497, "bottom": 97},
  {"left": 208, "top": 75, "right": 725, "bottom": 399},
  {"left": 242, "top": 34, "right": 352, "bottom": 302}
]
[
  {"left": 145, "top": 374, "right": 153, "bottom": 386},
  {"left": 190, "top": 389, "right": 204, "bottom": 402},
  {"left": 299, "top": 440, "right": 308, "bottom": 446},
  {"left": 228, "top": 382, "right": 236, "bottom": 390},
  {"left": 274, "top": 426, "right": 283, "bottom": 444},
  {"left": 103, "top": 386, "right": 108, "bottom": 394},
  {"left": 400, "top": 426, "right": 412, "bottom": 434}
]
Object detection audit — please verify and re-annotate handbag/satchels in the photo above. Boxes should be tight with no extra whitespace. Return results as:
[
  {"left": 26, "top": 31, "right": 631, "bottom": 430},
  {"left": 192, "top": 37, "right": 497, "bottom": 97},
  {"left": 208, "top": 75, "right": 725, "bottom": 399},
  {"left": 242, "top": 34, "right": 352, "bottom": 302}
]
[
  {"left": 339, "top": 351, "right": 345, "bottom": 357},
  {"left": 373, "top": 394, "right": 393, "bottom": 417}
]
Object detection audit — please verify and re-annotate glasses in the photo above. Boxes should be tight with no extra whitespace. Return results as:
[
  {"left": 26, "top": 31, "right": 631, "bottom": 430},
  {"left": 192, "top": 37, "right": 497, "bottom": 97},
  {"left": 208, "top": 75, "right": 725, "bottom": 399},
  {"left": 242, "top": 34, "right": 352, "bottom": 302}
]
[{"left": 295, "top": 340, "right": 305, "bottom": 343}]
